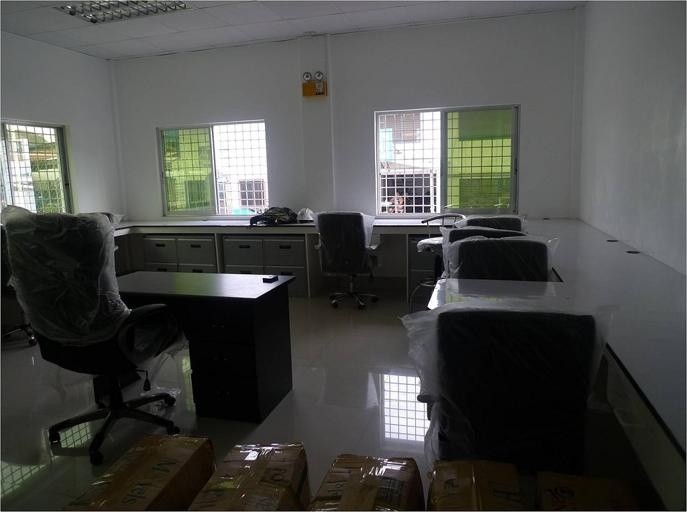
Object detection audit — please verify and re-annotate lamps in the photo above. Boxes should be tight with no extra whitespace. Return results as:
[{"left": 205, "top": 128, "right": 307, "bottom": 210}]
[{"left": 302, "top": 71, "right": 326, "bottom": 96}]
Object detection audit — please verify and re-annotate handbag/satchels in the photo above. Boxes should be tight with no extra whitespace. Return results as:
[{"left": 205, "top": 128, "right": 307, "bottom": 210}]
[{"left": 250, "top": 207, "right": 297, "bottom": 226}]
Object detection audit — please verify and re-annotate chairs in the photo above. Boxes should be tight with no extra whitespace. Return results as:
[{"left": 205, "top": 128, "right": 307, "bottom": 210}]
[
  {"left": 430, "top": 305, "right": 601, "bottom": 467},
  {"left": 315, "top": 212, "right": 380, "bottom": 311},
  {"left": 407, "top": 213, "right": 465, "bottom": 313},
  {"left": 2, "top": 209, "right": 182, "bottom": 464},
  {"left": 446, "top": 215, "right": 549, "bottom": 283}
]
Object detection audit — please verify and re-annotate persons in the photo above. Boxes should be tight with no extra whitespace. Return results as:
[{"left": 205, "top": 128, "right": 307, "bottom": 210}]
[{"left": 387, "top": 192, "right": 405, "bottom": 213}]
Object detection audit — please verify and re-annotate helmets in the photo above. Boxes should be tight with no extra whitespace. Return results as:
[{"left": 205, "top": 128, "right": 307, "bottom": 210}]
[{"left": 297, "top": 208, "right": 314, "bottom": 220}]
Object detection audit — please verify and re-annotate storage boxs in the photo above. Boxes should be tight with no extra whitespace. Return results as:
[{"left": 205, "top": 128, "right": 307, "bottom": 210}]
[
  {"left": 186, "top": 441, "right": 313, "bottom": 510},
  {"left": 427, "top": 459, "right": 535, "bottom": 509},
  {"left": 535, "top": 470, "right": 635, "bottom": 508},
  {"left": 61, "top": 431, "right": 216, "bottom": 510},
  {"left": 312, "top": 452, "right": 426, "bottom": 510}
]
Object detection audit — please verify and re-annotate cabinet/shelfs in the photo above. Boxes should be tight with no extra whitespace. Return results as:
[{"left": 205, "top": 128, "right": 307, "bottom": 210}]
[
  {"left": 144, "top": 233, "right": 217, "bottom": 274},
  {"left": 218, "top": 233, "right": 308, "bottom": 297}
]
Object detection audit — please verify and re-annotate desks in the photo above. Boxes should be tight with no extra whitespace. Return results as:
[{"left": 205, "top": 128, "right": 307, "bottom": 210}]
[
  {"left": 113, "top": 269, "right": 298, "bottom": 423},
  {"left": 310, "top": 211, "right": 687, "bottom": 508}
]
